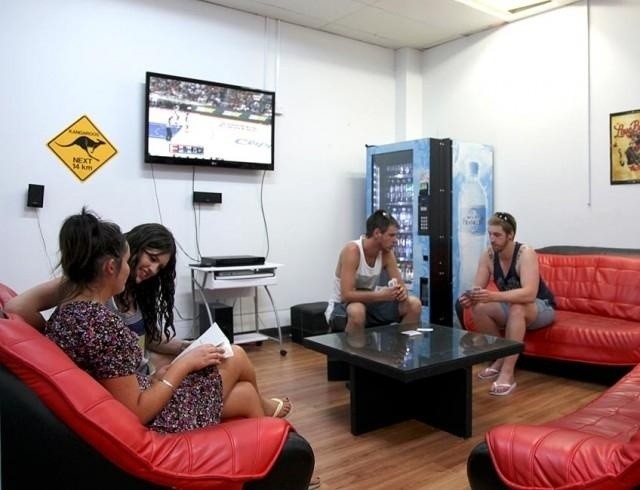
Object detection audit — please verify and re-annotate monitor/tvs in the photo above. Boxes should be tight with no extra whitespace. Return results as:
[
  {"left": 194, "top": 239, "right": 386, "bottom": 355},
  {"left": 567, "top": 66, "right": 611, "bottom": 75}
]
[{"left": 144, "top": 71, "right": 276, "bottom": 172}]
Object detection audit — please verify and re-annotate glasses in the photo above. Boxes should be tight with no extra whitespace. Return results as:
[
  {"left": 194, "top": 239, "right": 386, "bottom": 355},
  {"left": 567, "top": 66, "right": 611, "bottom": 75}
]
[
  {"left": 495, "top": 211, "right": 516, "bottom": 232},
  {"left": 382, "top": 210, "right": 393, "bottom": 219}
]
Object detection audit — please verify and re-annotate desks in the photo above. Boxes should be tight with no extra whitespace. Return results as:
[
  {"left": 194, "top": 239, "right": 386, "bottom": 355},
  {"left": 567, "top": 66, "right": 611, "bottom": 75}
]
[{"left": 187, "top": 261, "right": 287, "bottom": 359}]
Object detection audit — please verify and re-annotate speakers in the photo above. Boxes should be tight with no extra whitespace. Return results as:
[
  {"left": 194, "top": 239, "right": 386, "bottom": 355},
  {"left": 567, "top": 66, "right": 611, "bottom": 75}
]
[
  {"left": 199, "top": 302, "right": 234, "bottom": 344},
  {"left": 26, "top": 184, "right": 44, "bottom": 208}
]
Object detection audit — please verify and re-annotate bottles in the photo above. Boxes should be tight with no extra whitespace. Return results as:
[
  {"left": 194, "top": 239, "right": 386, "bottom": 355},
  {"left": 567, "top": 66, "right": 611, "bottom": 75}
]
[
  {"left": 407, "top": 207, "right": 412, "bottom": 234},
  {"left": 396, "top": 262, "right": 414, "bottom": 285},
  {"left": 385, "top": 186, "right": 412, "bottom": 204},
  {"left": 385, "top": 165, "right": 413, "bottom": 185},
  {"left": 400, "top": 209, "right": 408, "bottom": 233},
  {"left": 392, "top": 235, "right": 400, "bottom": 259},
  {"left": 405, "top": 232, "right": 406, "bottom": 233},
  {"left": 399, "top": 234, "right": 407, "bottom": 258},
  {"left": 392, "top": 208, "right": 401, "bottom": 229},
  {"left": 458, "top": 160, "right": 490, "bottom": 299},
  {"left": 407, "top": 237, "right": 415, "bottom": 260}
]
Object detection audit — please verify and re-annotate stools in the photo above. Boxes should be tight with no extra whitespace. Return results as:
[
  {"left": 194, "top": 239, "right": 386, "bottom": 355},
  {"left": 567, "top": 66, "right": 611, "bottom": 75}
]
[
  {"left": 327, "top": 305, "right": 405, "bottom": 381},
  {"left": 290, "top": 301, "right": 328, "bottom": 346}
]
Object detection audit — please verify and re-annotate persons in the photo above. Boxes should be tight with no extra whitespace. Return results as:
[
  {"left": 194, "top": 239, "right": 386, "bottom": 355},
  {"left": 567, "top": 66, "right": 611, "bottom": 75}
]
[
  {"left": 49, "top": 214, "right": 321, "bottom": 490},
  {"left": 8, "top": 224, "right": 208, "bottom": 380},
  {"left": 459, "top": 212, "right": 556, "bottom": 400},
  {"left": 324, "top": 211, "right": 425, "bottom": 338}
]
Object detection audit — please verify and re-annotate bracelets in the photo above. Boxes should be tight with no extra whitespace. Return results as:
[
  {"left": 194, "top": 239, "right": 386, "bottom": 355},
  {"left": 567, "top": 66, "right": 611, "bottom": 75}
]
[{"left": 154, "top": 368, "right": 176, "bottom": 392}]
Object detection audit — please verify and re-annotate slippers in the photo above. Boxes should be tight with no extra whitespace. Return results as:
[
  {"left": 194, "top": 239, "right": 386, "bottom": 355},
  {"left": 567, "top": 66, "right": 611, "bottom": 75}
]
[
  {"left": 268, "top": 397, "right": 294, "bottom": 416},
  {"left": 489, "top": 377, "right": 516, "bottom": 395},
  {"left": 477, "top": 367, "right": 498, "bottom": 379}
]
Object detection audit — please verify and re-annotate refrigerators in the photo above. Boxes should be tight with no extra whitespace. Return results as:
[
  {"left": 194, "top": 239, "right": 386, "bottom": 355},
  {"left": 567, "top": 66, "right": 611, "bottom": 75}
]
[{"left": 365, "top": 137, "right": 495, "bottom": 327}]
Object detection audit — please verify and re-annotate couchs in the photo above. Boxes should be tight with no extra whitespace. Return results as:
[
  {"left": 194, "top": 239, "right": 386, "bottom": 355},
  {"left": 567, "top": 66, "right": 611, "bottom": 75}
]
[
  {"left": 467, "top": 361, "right": 640, "bottom": 489},
  {"left": 456, "top": 244, "right": 640, "bottom": 384},
  {"left": 0, "top": 282, "right": 315, "bottom": 490}
]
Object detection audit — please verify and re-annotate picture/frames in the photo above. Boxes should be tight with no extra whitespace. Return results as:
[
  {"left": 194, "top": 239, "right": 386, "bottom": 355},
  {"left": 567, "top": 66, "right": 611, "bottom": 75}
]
[{"left": 610, "top": 108, "right": 640, "bottom": 184}]
